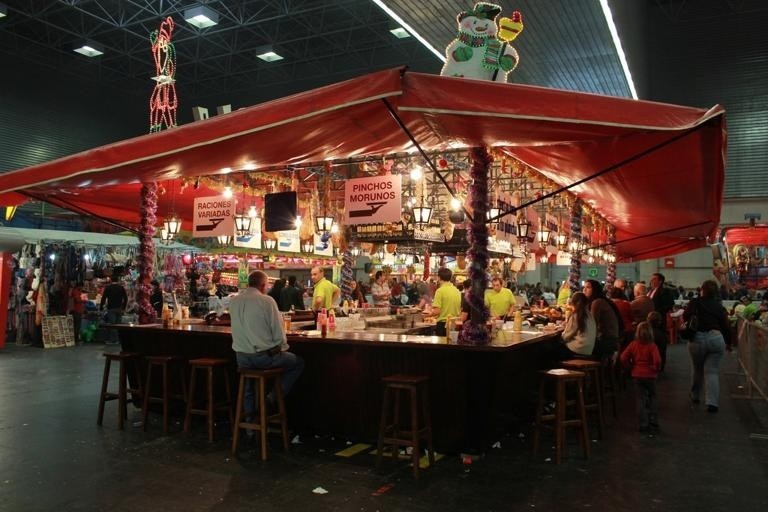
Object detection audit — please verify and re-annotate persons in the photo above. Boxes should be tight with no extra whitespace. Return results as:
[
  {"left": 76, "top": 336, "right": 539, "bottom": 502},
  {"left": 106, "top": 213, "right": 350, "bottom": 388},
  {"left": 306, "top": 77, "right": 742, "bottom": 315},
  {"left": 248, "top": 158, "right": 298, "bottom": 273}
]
[
  {"left": 518, "top": 272, "right": 733, "bottom": 439},
  {"left": 69, "top": 287, "right": 88, "bottom": 346},
  {"left": 149, "top": 280, "right": 163, "bottom": 317},
  {"left": 229, "top": 270, "right": 307, "bottom": 436},
  {"left": 100, "top": 276, "right": 128, "bottom": 345},
  {"left": 266, "top": 266, "right": 516, "bottom": 336}
]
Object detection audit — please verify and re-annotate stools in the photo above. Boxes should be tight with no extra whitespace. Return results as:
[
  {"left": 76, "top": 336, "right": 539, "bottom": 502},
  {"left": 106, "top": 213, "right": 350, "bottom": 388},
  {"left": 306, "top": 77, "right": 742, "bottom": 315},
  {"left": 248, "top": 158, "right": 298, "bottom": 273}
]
[
  {"left": 375, "top": 372, "right": 434, "bottom": 478},
  {"left": 98, "top": 351, "right": 150, "bottom": 431},
  {"left": 560, "top": 358, "right": 604, "bottom": 443},
  {"left": 231, "top": 366, "right": 290, "bottom": 461},
  {"left": 184, "top": 357, "right": 235, "bottom": 444},
  {"left": 142, "top": 353, "right": 188, "bottom": 436},
  {"left": 533, "top": 368, "right": 590, "bottom": 467}
]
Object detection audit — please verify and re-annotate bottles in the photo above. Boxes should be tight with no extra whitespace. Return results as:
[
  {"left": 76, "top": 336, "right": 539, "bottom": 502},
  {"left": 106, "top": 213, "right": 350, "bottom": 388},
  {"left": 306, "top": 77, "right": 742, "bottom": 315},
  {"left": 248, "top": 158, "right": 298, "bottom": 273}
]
[
  {"left": 512, "top": 309, "right": 522, "bottom": 331},
  {"left": 343, "top": 299, "right": 355, "bottom": 314},
  {"left": 182, "top": 306, "right": 190, "bottom": 320},
  {"left": 763, "top": 253, "right": 768, "bottom": 267},
  {"left": 163, "top": 306, "right": 174, "bottom": 327},
  {"left": 449, "top": 318, "right": 456, "bottom": 332}
]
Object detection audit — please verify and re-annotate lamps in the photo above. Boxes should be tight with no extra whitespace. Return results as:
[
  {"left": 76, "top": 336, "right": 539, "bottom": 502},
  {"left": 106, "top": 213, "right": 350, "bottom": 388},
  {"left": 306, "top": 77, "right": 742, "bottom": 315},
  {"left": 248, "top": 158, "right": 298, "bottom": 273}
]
[
  {"left": 72, "top": 40, "right": 105, "bottom": 60},
  {"left": 255, "top": 44, "right": 284, "bottom": 64},
  {"left": 385, "top": 19, "right": 411, "bottom": 40},
  {"left": 184, "top": 5, "right": 220, "bottom": 30},
  {"left": 159, "top": 173, "right": 622, "bottom": 263}
]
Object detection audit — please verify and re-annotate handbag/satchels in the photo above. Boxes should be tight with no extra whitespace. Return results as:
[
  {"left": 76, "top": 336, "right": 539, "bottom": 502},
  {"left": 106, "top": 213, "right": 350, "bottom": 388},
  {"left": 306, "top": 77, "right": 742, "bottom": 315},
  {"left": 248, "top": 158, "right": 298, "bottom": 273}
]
[{"left": 681, "top": 316, "right": 698, "bottom": 340}]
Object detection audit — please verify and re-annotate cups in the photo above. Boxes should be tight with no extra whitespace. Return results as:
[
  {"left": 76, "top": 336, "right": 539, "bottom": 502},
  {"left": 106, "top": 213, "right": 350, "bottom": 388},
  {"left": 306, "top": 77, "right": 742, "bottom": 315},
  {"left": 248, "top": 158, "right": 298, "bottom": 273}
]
[
  {"left": 495, "top": 320, "right": 504, "bottom": 330},
  {"left": 535, "top": 321, "right": 561, "bottom": 331},
  {"left": 283, "top": 315, "right": 292, "bottom": 330},
  {"left": 537, "top": 299, "right": 544, "bottom": 308},
  {"left": 450, "top": 331, "right": 460, "bottom": 343}
]
